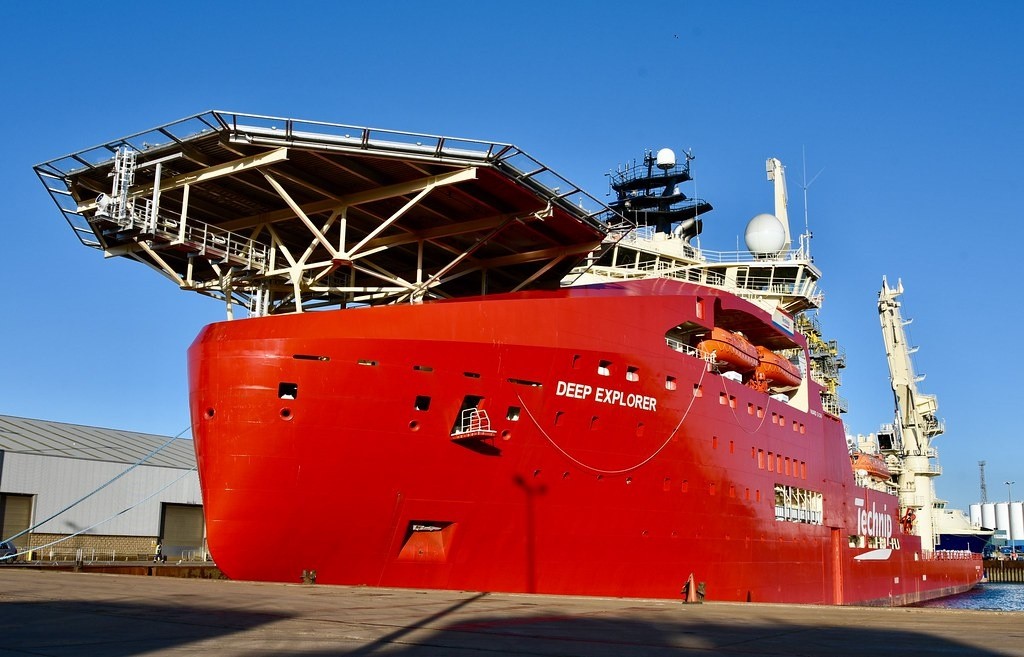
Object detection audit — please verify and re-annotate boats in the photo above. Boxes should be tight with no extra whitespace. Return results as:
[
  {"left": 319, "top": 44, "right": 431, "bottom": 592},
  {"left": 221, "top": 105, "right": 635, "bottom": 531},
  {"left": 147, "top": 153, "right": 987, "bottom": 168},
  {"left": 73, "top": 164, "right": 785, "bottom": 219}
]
[
  {"left": 744, "top": 346, "right": 801, "bottom": 387},
  {"left": 31, "top": 109, "right": 995, "bottom": 604},
  {"left": 693, "top": 327, "right": 758, "bottom": 373}
]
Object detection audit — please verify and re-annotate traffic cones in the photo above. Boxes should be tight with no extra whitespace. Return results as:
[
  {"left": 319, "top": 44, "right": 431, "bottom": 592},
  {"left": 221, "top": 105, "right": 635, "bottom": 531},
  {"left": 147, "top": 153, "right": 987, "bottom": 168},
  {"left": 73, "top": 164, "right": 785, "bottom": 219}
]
[{"left": 682, "top": 574, "right": 701, "bottom": 605}]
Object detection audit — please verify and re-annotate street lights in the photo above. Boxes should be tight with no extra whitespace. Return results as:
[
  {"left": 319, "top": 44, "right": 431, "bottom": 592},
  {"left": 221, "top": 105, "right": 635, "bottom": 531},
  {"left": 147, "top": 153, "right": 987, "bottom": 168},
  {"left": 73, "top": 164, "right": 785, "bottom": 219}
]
[{"left": 1005, "top": 482, "right": 1015, "bottom": 502}]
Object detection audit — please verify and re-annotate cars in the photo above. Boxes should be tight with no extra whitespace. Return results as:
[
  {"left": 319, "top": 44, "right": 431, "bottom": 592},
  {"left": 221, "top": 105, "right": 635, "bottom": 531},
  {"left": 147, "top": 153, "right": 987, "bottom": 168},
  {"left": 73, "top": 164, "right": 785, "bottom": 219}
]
[{"left": 0, "top": 541, "right": 17, "bottom": 564}]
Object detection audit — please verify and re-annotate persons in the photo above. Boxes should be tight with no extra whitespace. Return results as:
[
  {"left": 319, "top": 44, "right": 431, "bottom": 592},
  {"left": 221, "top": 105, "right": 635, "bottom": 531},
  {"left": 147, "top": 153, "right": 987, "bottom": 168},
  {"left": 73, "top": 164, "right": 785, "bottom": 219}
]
[
  {"left": 710, "top": 349, "right": 717, "bottom": 363},
  {"left": 606, "top": 221, "right": 612, "bottom": 232},
  {"left": 153, "top": 542, "right": 164, "bottom": 564}
]
